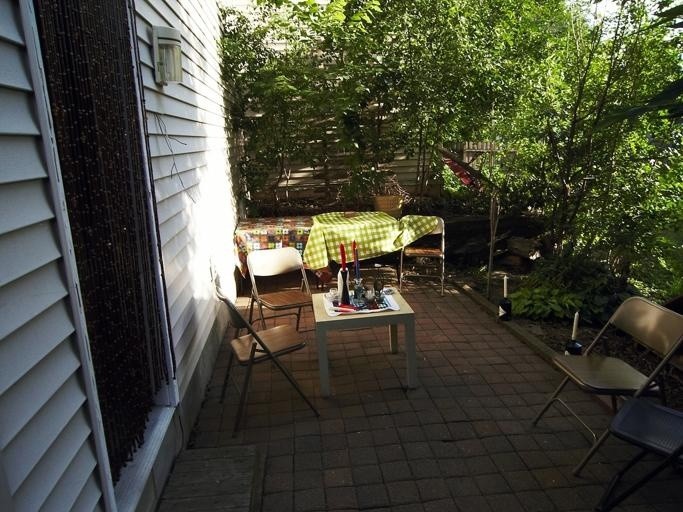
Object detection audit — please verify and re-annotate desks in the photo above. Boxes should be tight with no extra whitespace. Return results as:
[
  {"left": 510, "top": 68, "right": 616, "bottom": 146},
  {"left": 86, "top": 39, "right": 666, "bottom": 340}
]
[{"left": 311, "top": 286, "right": 420, "bottom": 398}]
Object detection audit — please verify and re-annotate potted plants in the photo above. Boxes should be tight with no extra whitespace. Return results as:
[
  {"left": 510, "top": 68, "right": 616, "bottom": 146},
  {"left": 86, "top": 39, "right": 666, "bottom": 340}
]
[{"left": 371, "top": 170, "right": 403, "bottom": 220}]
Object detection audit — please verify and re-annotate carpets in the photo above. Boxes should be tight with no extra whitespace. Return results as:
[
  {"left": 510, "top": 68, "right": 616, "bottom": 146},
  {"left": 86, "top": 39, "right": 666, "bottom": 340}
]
[{"left": 158, "top": 441, "right": 269, "bottom": 512}]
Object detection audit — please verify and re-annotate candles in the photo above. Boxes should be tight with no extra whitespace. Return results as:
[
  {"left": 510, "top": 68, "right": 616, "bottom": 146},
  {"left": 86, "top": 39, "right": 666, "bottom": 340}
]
[
  {"left": 355, "top": 248, "right": 360, "bottom": 281},
  {"left": 572, "top": 310, "right": 581, "bottom": 341},
  {"left": 340, "top": 244, "right": 346, "bottom": 272},
  {"left": 352, "top": 240, "right": 357, "bottom": 266},
  {"left": 504, "top": 275, "right": 508, "bottom": 298}
]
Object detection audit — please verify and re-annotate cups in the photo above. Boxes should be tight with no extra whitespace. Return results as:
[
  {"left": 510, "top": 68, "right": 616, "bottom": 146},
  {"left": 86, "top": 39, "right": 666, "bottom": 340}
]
[{"left": 330, "top": 288, "right": 336, "bottom": 295}]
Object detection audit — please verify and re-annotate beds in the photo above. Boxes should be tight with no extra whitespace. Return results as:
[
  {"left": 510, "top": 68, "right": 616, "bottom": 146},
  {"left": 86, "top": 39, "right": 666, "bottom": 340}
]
[{"left": 234, "top": 211, "right": 399, "bottom": 295}]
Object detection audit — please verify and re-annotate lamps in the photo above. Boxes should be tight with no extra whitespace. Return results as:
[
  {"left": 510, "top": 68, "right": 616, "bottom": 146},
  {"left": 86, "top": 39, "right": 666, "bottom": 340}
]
[{"left": 152, "top": 25, "right": 184, "bottom": 88}]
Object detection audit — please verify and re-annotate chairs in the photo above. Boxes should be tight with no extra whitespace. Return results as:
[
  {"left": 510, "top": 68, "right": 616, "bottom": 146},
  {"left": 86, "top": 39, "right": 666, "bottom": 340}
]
[
  {"left": 531, "top": 296, "right": 683, "bottom": 476},
  {"left": 247, "top": 246, "right": 314, "bottom": 333},
  {"left": 596, "top": 395, "right": 683, "bottom": 512},
  {"left": 214, "top": 275, "right": 321, "bottom": 438},
  {"left": 399, "top": 215, "right": 447, "bottom": 297}
]
